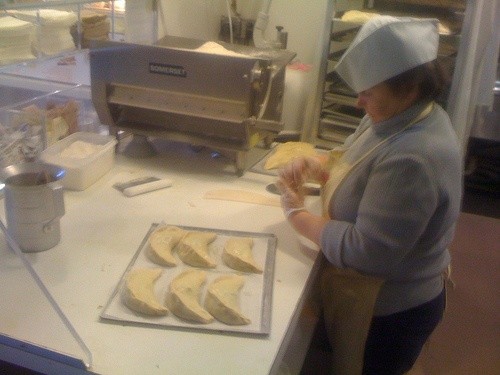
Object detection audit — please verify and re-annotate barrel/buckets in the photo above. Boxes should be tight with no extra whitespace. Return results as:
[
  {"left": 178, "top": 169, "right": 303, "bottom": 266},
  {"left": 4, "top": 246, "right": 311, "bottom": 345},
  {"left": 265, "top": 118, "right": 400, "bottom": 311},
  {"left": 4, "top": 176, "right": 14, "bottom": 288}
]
[{"left": 0, "top": 163, "right": 67, "bottom": 252}]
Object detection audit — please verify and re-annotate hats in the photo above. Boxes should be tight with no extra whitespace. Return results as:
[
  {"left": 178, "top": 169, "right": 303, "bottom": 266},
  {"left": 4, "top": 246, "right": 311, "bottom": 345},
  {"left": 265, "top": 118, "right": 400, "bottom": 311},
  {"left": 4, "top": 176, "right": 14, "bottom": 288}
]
[{"left": 334, "top": 16, "right": 440, "bottom": 93}]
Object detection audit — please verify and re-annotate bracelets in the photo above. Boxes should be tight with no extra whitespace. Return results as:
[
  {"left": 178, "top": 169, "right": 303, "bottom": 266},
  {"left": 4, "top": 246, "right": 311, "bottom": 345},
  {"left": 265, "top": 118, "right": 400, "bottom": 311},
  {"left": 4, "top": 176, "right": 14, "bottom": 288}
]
[{"left": 283, "top": 207, "right": 308, "bottom": 220}]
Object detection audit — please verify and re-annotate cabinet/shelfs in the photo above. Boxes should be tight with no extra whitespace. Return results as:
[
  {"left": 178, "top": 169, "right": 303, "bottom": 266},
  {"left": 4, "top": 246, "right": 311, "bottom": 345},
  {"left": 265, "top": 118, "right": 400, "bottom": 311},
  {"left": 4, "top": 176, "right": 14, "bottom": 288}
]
[{"left": 301, "top": 0, "right": 484, "bottom": 166}]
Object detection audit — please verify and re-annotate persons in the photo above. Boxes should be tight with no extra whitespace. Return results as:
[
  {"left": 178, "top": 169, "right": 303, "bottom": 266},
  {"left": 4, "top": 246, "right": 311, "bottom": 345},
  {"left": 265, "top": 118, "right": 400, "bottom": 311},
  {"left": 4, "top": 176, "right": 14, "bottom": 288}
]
[{"left": 274, "top": 14, "right": 463, "bottom": 375}]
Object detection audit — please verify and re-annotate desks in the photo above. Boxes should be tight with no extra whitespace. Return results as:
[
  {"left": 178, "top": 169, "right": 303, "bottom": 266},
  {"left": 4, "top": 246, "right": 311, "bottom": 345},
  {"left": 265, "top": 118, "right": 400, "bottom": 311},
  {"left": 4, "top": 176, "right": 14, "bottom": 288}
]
[{"left": 0, "top": 131, "right": 326, "bottom": 375}]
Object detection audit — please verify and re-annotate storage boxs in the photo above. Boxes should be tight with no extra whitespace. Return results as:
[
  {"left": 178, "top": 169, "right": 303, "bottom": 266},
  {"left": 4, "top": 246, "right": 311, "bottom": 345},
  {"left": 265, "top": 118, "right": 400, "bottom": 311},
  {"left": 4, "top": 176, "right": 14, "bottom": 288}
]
[{"left": 39, "top": 131, "right": 118, "bottom": 191}]
